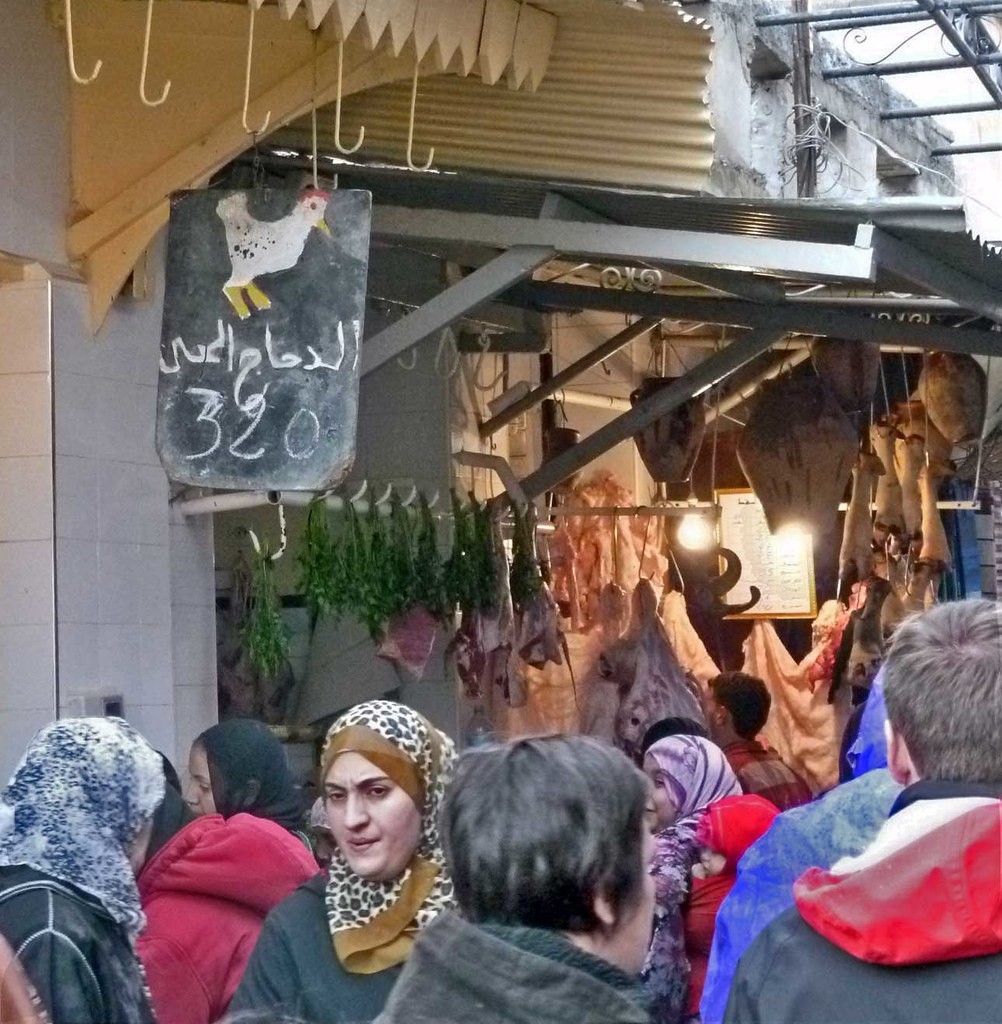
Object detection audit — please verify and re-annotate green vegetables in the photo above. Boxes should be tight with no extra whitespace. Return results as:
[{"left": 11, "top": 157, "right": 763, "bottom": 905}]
[{"left": 225, "top": 485, "right": 546, "bottom": 681}]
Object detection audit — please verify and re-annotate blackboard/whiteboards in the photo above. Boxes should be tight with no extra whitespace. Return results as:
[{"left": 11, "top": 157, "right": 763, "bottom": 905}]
[{"left": 154, "top": 191, "right": 372, "bottom": 488}]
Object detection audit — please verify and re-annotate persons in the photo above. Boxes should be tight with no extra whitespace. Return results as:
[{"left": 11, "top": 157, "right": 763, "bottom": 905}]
[
  {"left": 627, "top": 669, "right": 812, "bottom": 1024},
  {"left": 223, "top": 701, "right": 463, "bottom": 1022},
  {"left": 1, "top": 717, "right": 336, "bottom": 1024},
  {"left": 699, "top": 598, "right": 1002, "bottom": 1023},
  {"left": 372, "top": 731, "right": 661, "bottom": 1024}
]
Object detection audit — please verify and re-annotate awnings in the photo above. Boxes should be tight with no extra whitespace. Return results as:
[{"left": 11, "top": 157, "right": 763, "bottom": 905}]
[{"left": 270, "top": 150, "right": 1002, "bottom": 515}]
